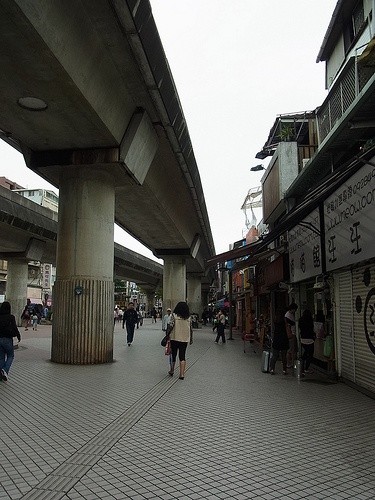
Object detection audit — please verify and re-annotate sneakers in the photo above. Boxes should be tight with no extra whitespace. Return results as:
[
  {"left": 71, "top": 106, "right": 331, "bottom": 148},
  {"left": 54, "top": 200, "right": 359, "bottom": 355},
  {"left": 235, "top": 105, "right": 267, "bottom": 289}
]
[{"left": 0, "top": 369, "right": 9, "bottom": 381}]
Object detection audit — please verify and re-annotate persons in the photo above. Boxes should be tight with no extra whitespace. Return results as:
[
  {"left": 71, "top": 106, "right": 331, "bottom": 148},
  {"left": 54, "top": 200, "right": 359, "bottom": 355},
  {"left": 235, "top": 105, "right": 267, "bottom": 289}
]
[
  {"left": 296, "top": 310, "right": 317, "bottom": 377},
  {"left": 0, "top": 301, "right": 21, "bottom": 381},
  {"left": 191, "top": 306, "right": 229, "bottom": 344},
  {"left": 21, "top": 305, "right": 43, "bottom": 331},
  {"left": 269, "top": 310, "right": 295, "bottom": 375},
  {"left": 167, "top": 301, "right": 193, "bottom": 379},
  {"left": 122, "top": 302, "right": 139, "bottom": 347},
  {"left": 162, "top": 308, "right": 173, "bottom": 331},
  {"left": 285, "top": 303, "right": 298, "bottom": 368},
  {"left": 114, "top": 305, "right": 161, "bottom": 328}
]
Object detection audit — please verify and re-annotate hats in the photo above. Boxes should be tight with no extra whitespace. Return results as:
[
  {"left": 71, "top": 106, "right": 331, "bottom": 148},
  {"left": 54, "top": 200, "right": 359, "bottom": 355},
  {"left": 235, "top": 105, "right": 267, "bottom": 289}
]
[{"left": 167, "top": 307, "right": 171, "bottom": 311}]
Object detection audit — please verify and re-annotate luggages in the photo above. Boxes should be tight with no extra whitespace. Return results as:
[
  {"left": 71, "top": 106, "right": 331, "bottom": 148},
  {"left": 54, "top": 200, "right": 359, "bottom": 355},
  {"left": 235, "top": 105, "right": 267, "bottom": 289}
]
[{"left": 261, "top": 350, "right": 274, "bottom": 372}]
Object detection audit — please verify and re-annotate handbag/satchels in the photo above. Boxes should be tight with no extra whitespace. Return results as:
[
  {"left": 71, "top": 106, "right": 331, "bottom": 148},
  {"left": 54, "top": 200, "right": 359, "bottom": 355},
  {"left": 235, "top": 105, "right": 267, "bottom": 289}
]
[
  {"left": 161, "top": 336, "right": 168, "bottom": 346},
  {"left": 165, "top": 340, "right": 171, "bottom": 355}
]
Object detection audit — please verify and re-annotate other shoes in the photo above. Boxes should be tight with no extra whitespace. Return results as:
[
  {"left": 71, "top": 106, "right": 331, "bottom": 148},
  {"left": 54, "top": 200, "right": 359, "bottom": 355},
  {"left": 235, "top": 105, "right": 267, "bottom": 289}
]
[
  {"left": 282, "top": 370, "right": 287, "bottom": 375},
  {"left": 269, "top": 370, "right": 275, "bottom": 374},
  {"left": 169, "top": 370, "right": 174, "bottom": 376},
  {"left": 179, "top": 377, "right": 185, "bottom": 380}
]
